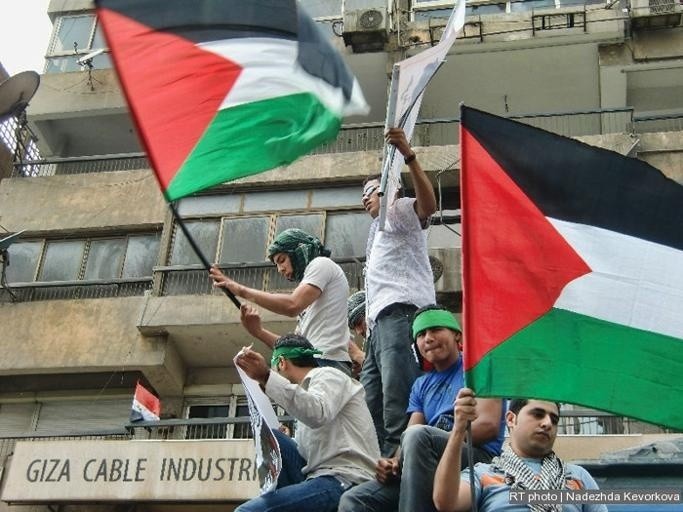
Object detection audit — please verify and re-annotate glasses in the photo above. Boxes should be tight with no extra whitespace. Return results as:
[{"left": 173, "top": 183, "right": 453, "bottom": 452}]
[{"left": 362, "top": 185, "right": 382, "bottom": 205}]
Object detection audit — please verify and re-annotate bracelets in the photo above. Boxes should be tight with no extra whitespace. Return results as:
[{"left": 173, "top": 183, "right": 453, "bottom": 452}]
[{"left": 404, "top": 153, "right": 417, "bottom": 165}]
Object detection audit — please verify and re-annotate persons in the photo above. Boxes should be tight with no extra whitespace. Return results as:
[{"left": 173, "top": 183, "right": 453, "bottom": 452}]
[
  {"left": 209, "top": 227, "right": 355, "bottom": 379},
  {"left": 234, "top": 342, "right": 283, "bottom": 496},
  {"left": 433, "top": 388, "right": 609, "bottom": 512},
  {"left": 360, "top": 128, "right": 436, "bottom": 459},
  {"left": 348, "top": 291, "right": 366, "bottom": 368},
  {"left": 234, "top": 333, "right": 382, "bottom": 512},
  {"left": 339, "top": 305, "right": 507, "bottom": 512}
]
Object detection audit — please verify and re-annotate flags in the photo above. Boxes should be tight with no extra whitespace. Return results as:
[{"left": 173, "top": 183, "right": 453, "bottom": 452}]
[
  {"left": 130, "top": 380, "right": 161, "bottom": 432},
  {"left": 92, "top": 0, "right": 372, "bottom": 204},
  {"left": 460, "top": 104, "right": 683, "bottom": 432},
  {"left": 378, "top": 1, "right": 466, "bottom": 233}
]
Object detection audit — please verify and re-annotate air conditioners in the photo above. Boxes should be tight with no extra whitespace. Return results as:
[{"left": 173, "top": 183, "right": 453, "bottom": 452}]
[
  {"left": 342, "top": 6, "right": 390, "bottom": 47},
  {"left": 627, "top": 0, "right": 683, "bottom": 32}
]
